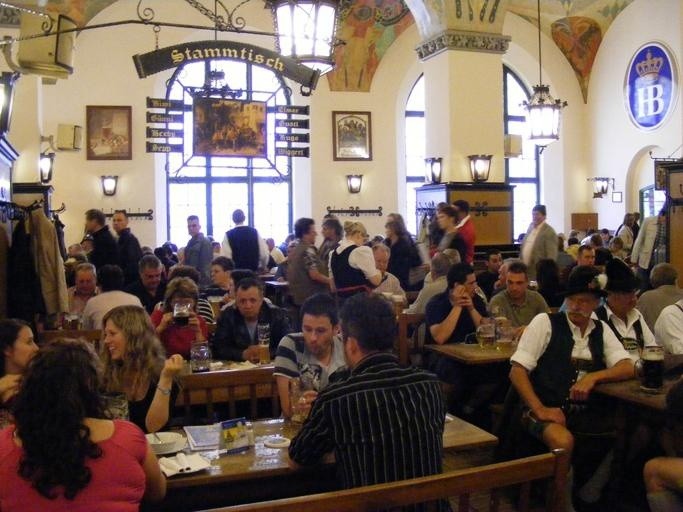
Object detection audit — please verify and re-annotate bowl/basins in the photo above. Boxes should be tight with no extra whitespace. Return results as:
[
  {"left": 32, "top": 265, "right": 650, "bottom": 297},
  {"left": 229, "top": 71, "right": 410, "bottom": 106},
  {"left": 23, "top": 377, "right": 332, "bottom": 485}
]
[{"left": 147, "top": 432, "right": 180, "bottom": 451}]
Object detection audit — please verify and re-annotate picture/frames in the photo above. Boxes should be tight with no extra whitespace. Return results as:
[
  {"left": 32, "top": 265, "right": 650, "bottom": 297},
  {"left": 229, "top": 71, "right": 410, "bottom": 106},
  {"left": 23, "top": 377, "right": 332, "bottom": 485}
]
[
  {"left": 86, "top": 105, "right": 132, "bottom": 160},
  {"left": 330, "top": 110, "right": 372, "bottom": 162}
]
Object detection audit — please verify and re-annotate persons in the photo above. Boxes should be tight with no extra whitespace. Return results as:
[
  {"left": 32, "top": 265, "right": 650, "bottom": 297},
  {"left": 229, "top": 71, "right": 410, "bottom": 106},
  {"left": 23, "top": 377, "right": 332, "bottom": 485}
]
[{"left": 1, "top": 199, "right": 682, "bottom": 511}]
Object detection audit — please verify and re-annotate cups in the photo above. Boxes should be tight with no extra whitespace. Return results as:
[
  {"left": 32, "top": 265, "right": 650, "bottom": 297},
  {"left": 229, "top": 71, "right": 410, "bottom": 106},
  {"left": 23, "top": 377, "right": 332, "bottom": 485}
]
[
  {"left": 172, "top": 303, "right": 190, "bottom": 328},
  {"left": 209, "top": 301, "right": 220, "bottom": 322},
  {"left": 288, "top": 386, "right": 309, "bottom": 419},
  {"left": 477, "top": 315, "right": 514, "bottom": 353},
  {"left": 190, "top": 338, "right": 209, "bottom": 374},
  {"left": 62, "top": 313, "right": 80, "bottom": 329},
  {"left": 258, "top": 344, "right": 270, "bottom": 364},
  {"left": 382, "top": 292, "right": 404, "bottom": 320},
  {"left": 633, "top": 347, "right": 665, "bottom": 393}
]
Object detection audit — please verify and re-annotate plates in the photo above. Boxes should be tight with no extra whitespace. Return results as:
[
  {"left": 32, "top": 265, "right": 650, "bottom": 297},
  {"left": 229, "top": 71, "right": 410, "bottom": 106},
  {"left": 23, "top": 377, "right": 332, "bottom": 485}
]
[{"left": 144, "top": 439, "right": 185, "bottom": 455}]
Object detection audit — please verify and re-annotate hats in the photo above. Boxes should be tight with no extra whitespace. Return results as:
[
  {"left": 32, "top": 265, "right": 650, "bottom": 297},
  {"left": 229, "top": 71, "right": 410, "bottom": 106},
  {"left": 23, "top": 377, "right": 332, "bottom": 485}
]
[{"left": 558, "top": 264, "right": 608, "bottom": 301}]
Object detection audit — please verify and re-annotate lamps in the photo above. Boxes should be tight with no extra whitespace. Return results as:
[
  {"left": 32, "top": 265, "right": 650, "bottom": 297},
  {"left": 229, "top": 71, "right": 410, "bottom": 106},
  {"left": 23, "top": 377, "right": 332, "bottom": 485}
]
[
  {"left": 100, "top": 173, "right": 120, "bottom": 197},
  {"left": 346, "top": 173, "right": 364, "bottom": 193},
  {"left": 261, "top": 0, "right": 342, "bottom": 76},
  {"left": 422, "top": 157, "right": 443, "bottom": 183},
  {"left": 588, "top": 176, "right": 607, "bottom": 199},
  {"left": 40, "top": 137, "right": 56, "bottom": 183},
  {"left": 468, "top": 153, "right": 494, "bottom": 182},
  {"left": 520, "top": 1, "right": 567, "bottom": 144}
]
